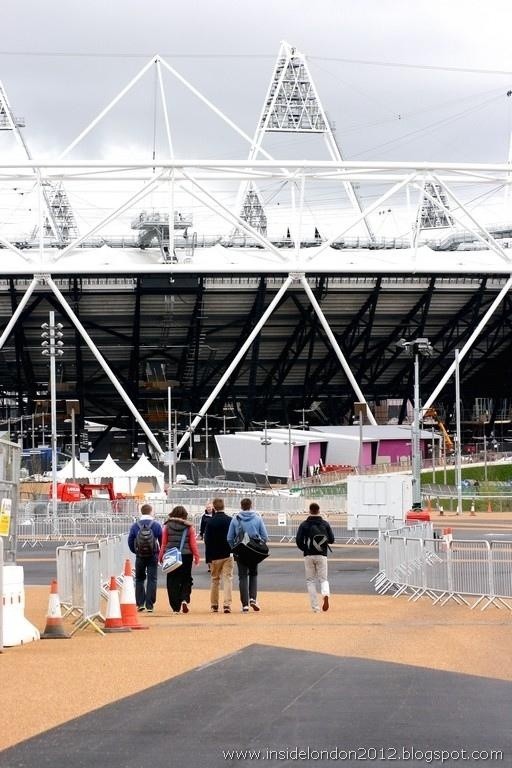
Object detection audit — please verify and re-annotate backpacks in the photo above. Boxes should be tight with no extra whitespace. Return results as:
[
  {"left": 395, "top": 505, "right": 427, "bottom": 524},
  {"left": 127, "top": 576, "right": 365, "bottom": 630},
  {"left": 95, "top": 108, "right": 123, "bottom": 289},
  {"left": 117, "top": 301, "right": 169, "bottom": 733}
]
[
  {"left": 311, "top": 524, "right": 329, "bottom": 548},
  {"left": 134, "top": 520, "right": 155, "bottom": 556}
]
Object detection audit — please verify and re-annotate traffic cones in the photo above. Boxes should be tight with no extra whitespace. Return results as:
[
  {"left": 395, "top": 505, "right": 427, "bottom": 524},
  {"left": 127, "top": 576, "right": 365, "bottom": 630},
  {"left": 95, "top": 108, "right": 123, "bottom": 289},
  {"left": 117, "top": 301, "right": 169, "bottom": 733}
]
[
  {"left": 469, "top": 503, "right": 476, "bottom": 516},
  {"left": 119, "top": 559, "right": 148, "bottom": 629},
  {"left": 439, "top": 529, "right": 451, "bottom": 553},
  {"left": 99, "top": 576, "right": 131, "bottom": 630},
  {"left": 447, "top": 528, "right": 455, "bottom": 547},
  {"left": 39, "top": 579, "right": 72, "bottom": 638},
  {"left": 487, "top": 502, "right": 491, "bottom": 512}
]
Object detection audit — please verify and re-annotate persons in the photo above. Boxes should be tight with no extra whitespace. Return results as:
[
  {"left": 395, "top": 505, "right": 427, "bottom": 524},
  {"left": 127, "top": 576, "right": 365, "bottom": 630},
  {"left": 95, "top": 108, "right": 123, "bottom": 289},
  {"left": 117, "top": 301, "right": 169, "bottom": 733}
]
[
  {"left": 227, "top": 498, "right": 268, "bottom": 612},
  {"left": 128, "top": 504, "right": 163, "bottom": 612},
  {"left": 296, "top": 503, "right": 334, "bottom": 613},
  {"left": 200, "top": 502, "right": 216, "bottom": 573},
  {"left": 205, "top": 497, "right": 237, "bottom": 614},
  {"left": 160, "top": 506, "right": 200, "bottom": 614}
]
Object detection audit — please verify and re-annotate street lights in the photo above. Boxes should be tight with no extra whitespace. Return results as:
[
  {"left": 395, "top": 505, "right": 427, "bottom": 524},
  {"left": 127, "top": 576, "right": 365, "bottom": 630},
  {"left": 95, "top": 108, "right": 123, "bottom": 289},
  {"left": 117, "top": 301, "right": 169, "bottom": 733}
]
[{"left": 0, "top": 313, "right": 464, "bottom": 534}]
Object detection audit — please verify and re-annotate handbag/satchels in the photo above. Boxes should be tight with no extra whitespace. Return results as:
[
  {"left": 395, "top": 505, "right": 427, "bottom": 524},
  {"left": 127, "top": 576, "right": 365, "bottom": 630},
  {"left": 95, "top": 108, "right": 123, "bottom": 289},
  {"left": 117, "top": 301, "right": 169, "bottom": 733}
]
[
  {"left": 161, "top": 547, "right": 183, "bottom": 574},
  {"left": 231, "top": 533, "right": 269, "bottom": 569}
]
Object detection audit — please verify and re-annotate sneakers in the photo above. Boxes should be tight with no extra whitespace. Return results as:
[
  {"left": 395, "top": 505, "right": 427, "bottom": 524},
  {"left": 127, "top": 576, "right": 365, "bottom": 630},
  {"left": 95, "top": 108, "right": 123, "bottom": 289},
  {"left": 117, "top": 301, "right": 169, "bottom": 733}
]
[
  {"left": 250, "top": 601, "right": 260, "bottom": 611},
  {"left": 323, "top": 595, "right": 329, "bottom": 611},
  {"left": 243, "top": 608, "right": 248, "bottom": 611},
  {"left": 137, "top": 604, "right": 145, "bottom": 611},
  {"left": 208, "top": 569, "right": 210, "bottom": 572},
  {"left": 173, "top": 611, "right": 179, "bottom": 615},
  {"left": 313, "top": 606, "right": 320, "bottom": 613},
  {"left": 224, "top": 606, "right": 231, "bottom": 613},
  {"left": 182, "top": 602, "right": 188, "bottom": 613},
  {"left": 211, "top": 605, "right": 218, "bottom": 612},
  {"left": 147, "top": 608, "right": 153, "bottom": 612}
]
